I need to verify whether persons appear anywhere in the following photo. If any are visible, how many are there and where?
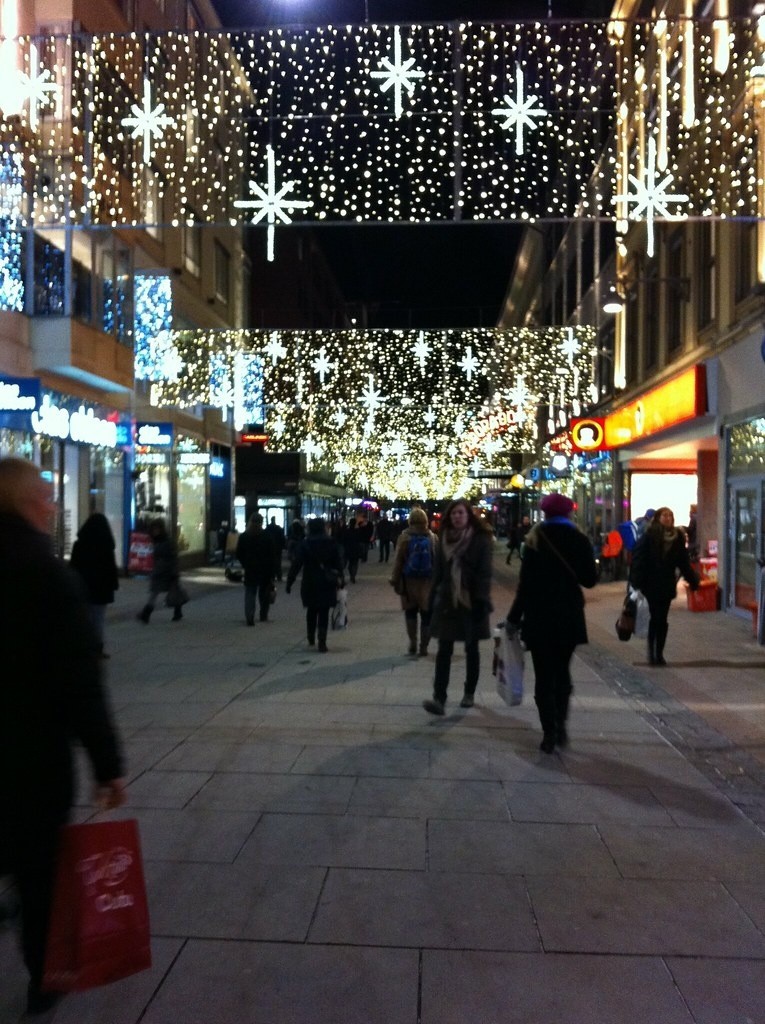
[506,493,598,752]
[70,513,120,658]
[138,517,182,625]
[630,508,697,665]
[219,503,699,652]
[0,458,130,1014]
[420,498,491,716]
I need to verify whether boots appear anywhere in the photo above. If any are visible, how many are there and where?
[533,685,572,755]
[646,619,669,667]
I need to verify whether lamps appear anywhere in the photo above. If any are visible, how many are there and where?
[602,277,690,313]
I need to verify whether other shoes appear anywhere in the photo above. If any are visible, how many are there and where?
[460,692,474,707]
[422,698,445,716]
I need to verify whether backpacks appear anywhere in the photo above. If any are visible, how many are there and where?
[402,532,434,579]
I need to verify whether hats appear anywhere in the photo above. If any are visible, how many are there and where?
[539,493,573,517]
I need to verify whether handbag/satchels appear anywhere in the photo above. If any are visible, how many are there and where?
[491,625,524,707]
[331,587,349,630]
[615,589,652,642]
[266,581,277,604]
[165,585,190,608]
[39,799,152,989]
